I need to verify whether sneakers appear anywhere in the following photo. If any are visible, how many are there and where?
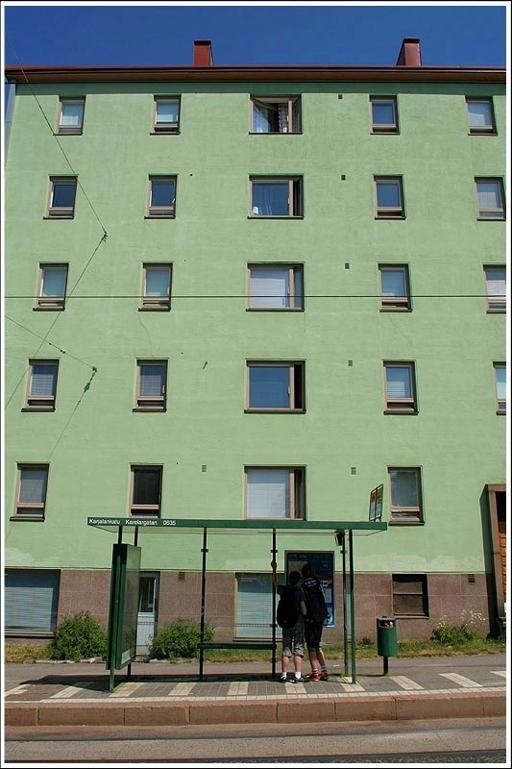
[277,674,329,683]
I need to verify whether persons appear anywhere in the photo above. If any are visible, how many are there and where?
[299,561,328,682]
[271,560,308,683]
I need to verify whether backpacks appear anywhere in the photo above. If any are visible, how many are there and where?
[303,582,328,622]
[277,587,299,629]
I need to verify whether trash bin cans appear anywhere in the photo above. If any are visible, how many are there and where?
[376,615,397,675]
